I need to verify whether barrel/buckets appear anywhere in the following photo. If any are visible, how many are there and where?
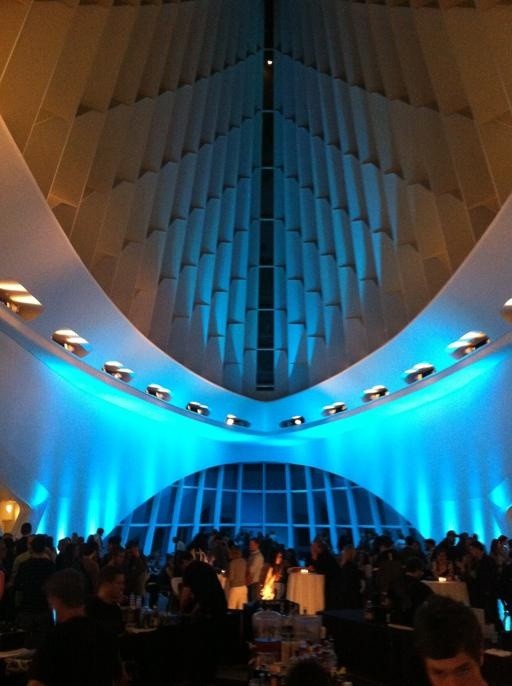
[294,615,321,642]
[253,610,282,642]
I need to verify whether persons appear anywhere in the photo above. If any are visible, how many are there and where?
[5,521,512,685]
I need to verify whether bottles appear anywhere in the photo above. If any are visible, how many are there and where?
[128,592,160,628]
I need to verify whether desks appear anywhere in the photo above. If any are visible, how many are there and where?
[423,580,470,607]
[289,573,325,615]
